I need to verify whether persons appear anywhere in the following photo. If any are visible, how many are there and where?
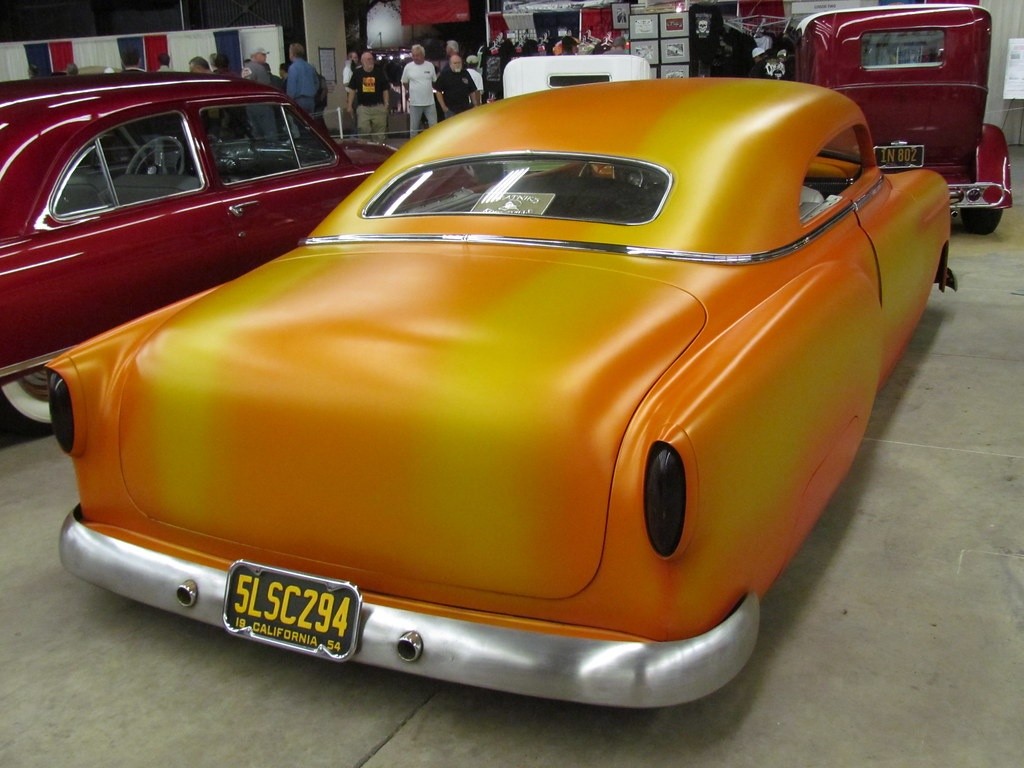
[348,52,390,144]
[121,48,145,73]
[603,37,626,54]
[29,64,40,78]
[68,65,78,75]
[190,52,246,140]
[158,54,173,72]
[240,43,328,141]
[560,37,580,55]
[435,41,478,123]
[401,45,437,139]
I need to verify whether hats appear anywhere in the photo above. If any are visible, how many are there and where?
[752,47,765,58]
[250,46,269,55]
[103,67,115,73]
[466,55,479,63]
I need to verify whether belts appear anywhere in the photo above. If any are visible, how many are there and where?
[360,101,384,107]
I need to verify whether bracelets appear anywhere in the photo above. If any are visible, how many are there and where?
[385,103,390,105]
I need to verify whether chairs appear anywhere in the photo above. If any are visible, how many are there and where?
[560,161,644,220]
[800,158,859,200]
[55,174,203,213]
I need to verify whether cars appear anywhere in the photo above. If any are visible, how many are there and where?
[788,3,1013,237]
[0,72,402,436]
[46,75,952,709]
